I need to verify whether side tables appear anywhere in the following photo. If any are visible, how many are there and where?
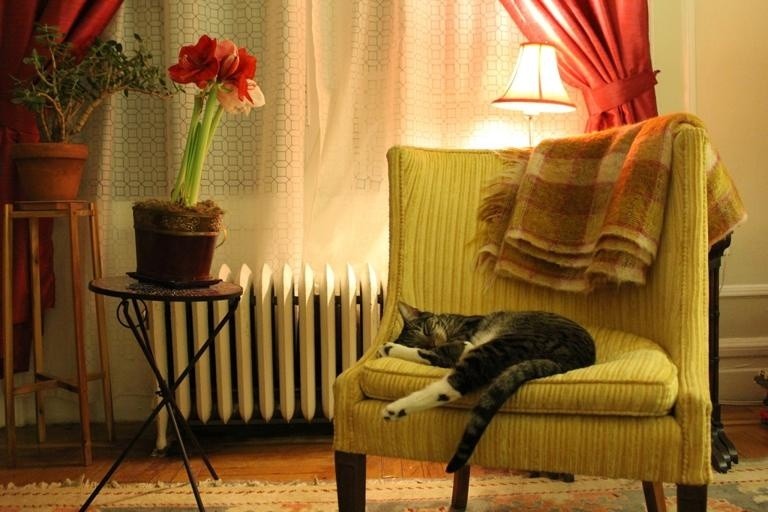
[77,276,243,512]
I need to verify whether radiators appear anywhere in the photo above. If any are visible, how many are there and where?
[146,263,387,451]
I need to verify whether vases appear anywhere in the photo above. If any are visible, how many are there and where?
[119,201,234,286]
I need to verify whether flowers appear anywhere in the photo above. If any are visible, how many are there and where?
[169,35,266,207]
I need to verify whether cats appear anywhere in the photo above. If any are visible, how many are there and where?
[376,300,596,473]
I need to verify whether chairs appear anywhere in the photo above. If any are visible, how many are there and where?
[331,122,712,512]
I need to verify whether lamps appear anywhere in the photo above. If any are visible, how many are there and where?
[491,42,576,149]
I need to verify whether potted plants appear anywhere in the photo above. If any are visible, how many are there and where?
[7,24,186,201]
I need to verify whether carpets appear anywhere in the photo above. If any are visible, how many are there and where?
[0,458,768,512]
